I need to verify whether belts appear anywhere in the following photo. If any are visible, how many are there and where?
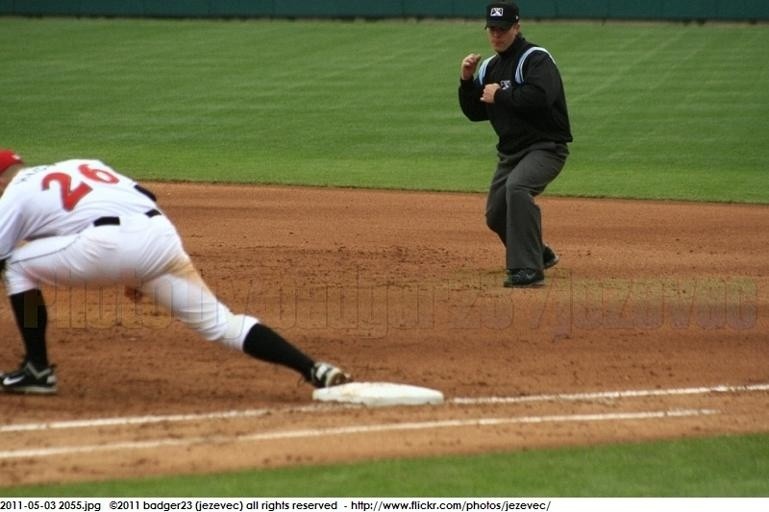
[85,208,164,230]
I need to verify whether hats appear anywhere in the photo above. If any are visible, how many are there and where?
[0,149,23,176]
[482,2,521,31]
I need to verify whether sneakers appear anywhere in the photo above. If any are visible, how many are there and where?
[308,361,353,388]
[504,243,559,275]
[502,267,545,288]
[0,361,60,394]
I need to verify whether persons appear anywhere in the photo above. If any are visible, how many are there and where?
[456,1,575,289]
[0,146,355,399]
